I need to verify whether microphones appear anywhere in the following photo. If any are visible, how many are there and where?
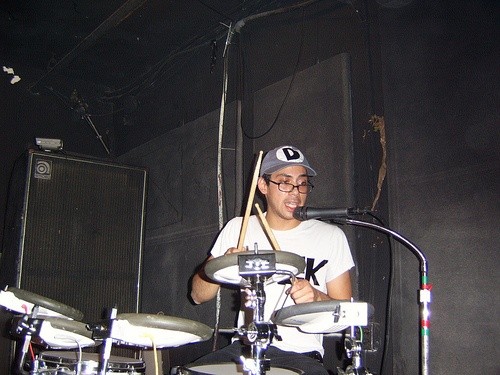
[292,206,371,221]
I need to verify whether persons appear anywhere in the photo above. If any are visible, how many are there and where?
[186,146,355,375]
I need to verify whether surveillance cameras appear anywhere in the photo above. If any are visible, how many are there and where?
[35,137,64,153]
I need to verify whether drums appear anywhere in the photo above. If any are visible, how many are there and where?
[203,250,306,288]
[36,351,146,375]
[113,313,213,348]
[0,287,84,320]
[179,362,305,375]
[11,315,103,348]
[271,301,375,333]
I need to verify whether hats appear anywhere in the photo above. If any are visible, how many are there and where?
[258,145,318,178]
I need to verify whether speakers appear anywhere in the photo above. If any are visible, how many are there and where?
[9,149,149,375]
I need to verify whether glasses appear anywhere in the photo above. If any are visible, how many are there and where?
[265,178,315,194]
